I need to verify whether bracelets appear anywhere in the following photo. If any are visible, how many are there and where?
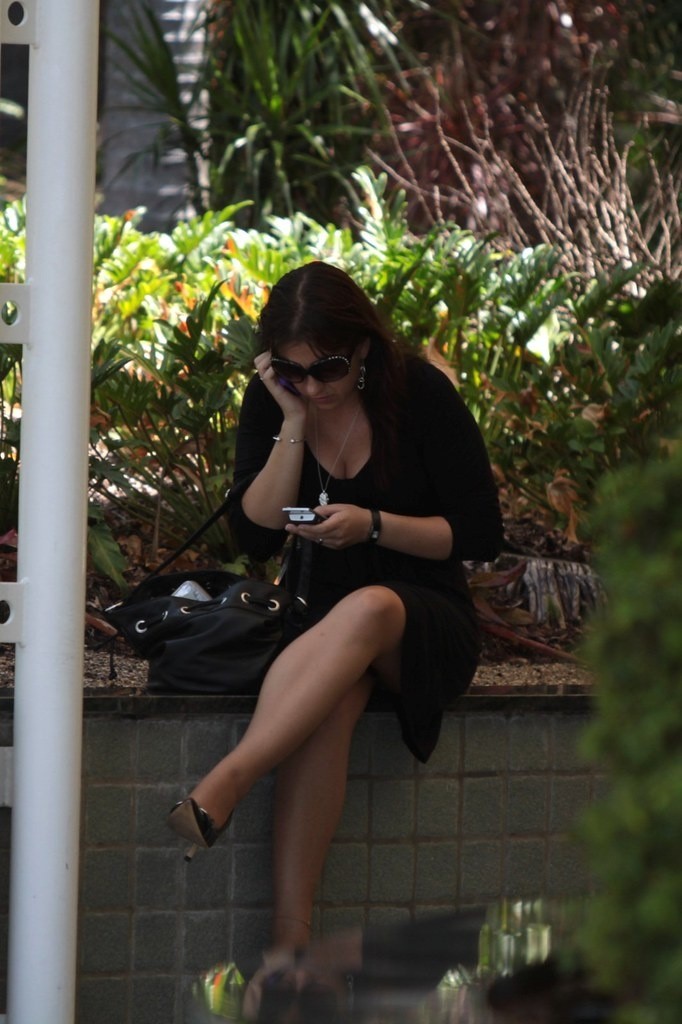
[272,435,308,444]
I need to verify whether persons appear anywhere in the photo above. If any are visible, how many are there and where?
[169,262,504,1024]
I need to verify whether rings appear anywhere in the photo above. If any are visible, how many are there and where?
[317,539,325,544]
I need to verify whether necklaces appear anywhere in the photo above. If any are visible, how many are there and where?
[313,402,362,507]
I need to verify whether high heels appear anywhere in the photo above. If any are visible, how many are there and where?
[166,797,234,863]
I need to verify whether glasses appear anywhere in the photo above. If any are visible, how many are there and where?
[270,339,361,384]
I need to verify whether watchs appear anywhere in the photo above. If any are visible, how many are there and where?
[364,507,382,552]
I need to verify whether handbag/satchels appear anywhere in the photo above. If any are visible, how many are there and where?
[102,472,321,695]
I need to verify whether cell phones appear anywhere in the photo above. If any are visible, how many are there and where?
[282,507,329,525]
[277,374,302,397]
